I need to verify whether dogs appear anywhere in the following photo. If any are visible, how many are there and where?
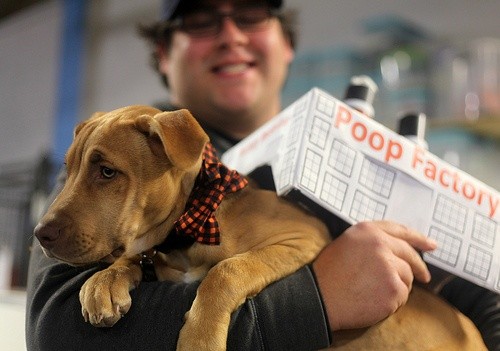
[31,103,489,351]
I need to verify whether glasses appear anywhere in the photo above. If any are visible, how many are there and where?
[173,6,278,36]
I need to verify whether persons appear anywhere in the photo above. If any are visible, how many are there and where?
[25,0,500,351]
